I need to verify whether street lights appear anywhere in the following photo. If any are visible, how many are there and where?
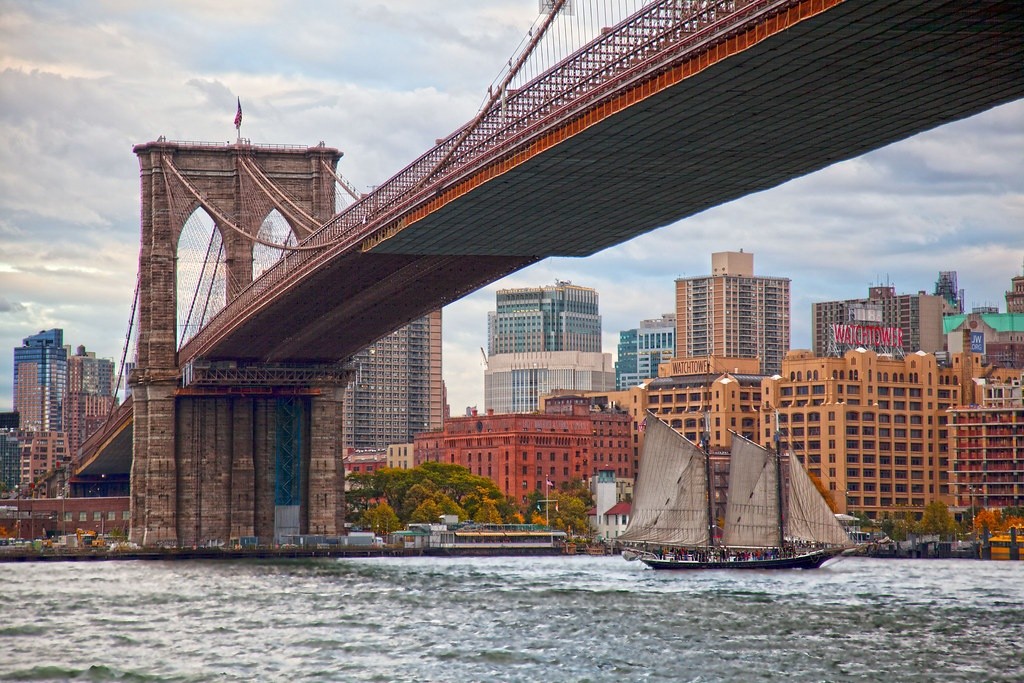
[967,485,978,529]
[15,483,20,538]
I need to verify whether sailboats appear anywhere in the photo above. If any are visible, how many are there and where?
[607,353,895,570]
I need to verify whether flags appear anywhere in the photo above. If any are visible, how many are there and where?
[234,101,242,129]
[547,480,553,486]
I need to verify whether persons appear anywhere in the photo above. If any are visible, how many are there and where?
[659,546,779,562]
[783,540,823,558]
